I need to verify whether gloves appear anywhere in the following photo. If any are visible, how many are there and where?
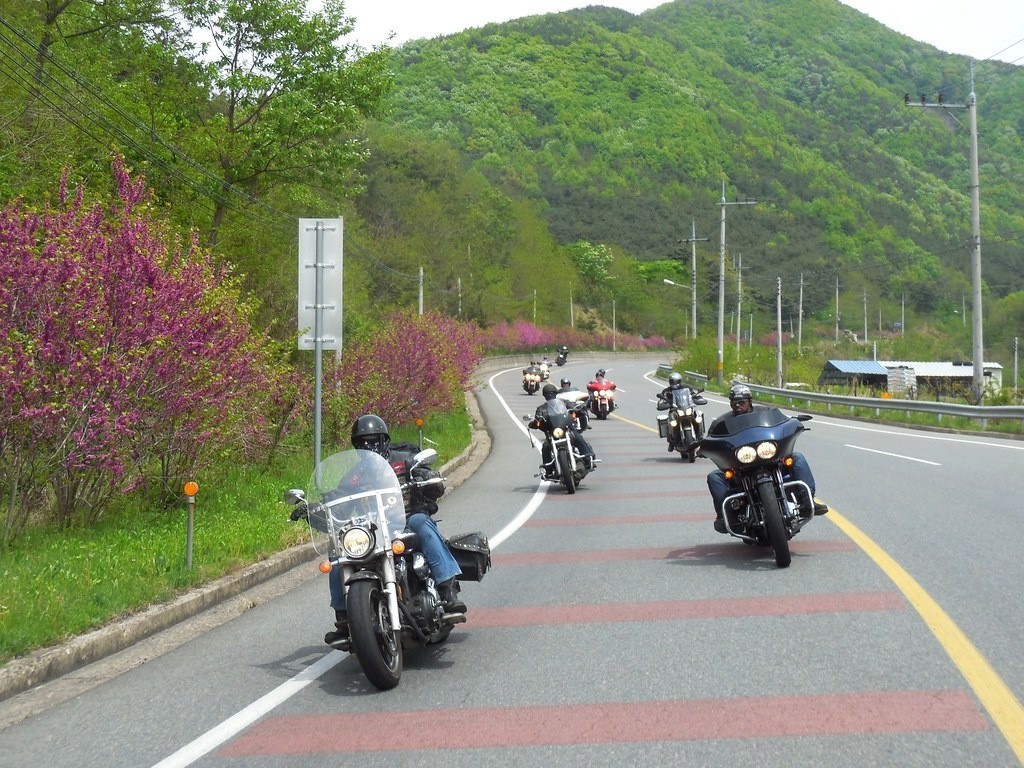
[425,471,441,481]
[290,504,308,522]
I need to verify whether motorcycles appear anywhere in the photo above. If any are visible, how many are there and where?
[521,399,602,494]
[584,376,618,420]
[283,419,493,691]
[689,408,818,568]
[554,348,570,366]
[553,386,594,436]
[522,369,544,395]
[655,386,708,463]
[536,361,553,382]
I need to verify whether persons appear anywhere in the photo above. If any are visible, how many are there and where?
[561,346,569,363]
[705,384,828,533]
[542,356,552,367]
[535,361,543,372]
[658,370,712,460]
[290,415,468,651]
[528,383,597,479]
[586,367,618,409]
[557,378,593,430]
[526,360,540,373]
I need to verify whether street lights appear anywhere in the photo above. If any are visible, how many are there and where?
[661,278,697,344]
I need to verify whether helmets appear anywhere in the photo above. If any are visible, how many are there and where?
[728,385,753,401]
[543,356,547,360]
[596,372,605,378]
[599,369,606,374]
[531,361,536,365]
[562,345,567,350]
[668,373,683,390]
[560,377,572,387]
[350,414,391,447]
[542,383,559,396]
[536,361,542,365]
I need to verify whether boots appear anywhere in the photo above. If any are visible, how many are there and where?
[667,439,675,452]
[540,468,560,481]
[438,576,467,613]
[798,493,828,518]
[324,610,350,644]
[714,506,745,534]
[583,457,597,470]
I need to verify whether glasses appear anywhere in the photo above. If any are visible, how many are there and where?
[732,400,748,405]
